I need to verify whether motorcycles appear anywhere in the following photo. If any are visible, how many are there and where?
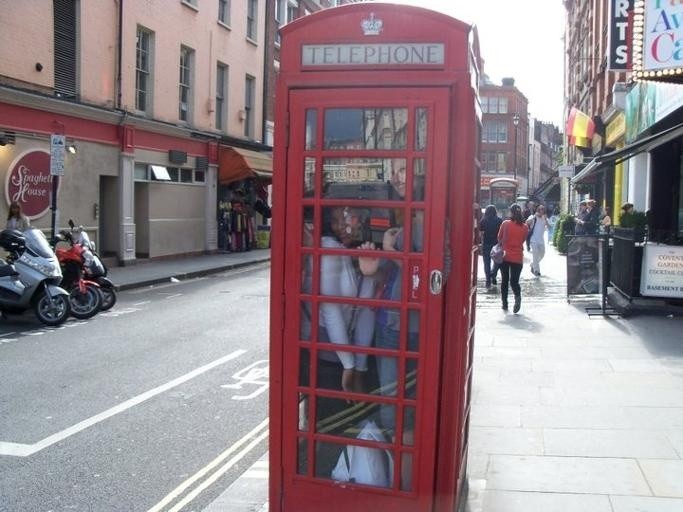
[0,218,117,326]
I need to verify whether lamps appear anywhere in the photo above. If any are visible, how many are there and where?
[65,143,77,154]
[0,132,16,146]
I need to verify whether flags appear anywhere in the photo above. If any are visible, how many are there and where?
[566,134,592,151]
[565,106,598,140]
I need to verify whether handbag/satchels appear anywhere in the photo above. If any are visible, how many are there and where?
[488,242,508,266]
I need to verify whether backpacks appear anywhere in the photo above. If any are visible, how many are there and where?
[330,421,396,490]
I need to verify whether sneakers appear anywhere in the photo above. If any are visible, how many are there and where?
[501,303,509,311]
[529,263,542,277]
[513,299,522,314]
[484,276,498,290]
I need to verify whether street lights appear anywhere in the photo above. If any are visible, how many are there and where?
[512,111,521,180]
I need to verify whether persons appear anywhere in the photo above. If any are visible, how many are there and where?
[573,201,589,235]
[598,205,611,229]
[524,202,552,278]
[521,199,535,252]
[298,183,381,474]
[579,199,602,236]
[371,140,452,495]
[478,203,503,289]
[496,203,529,313]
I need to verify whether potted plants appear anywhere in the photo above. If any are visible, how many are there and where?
[577,184,591,194]
